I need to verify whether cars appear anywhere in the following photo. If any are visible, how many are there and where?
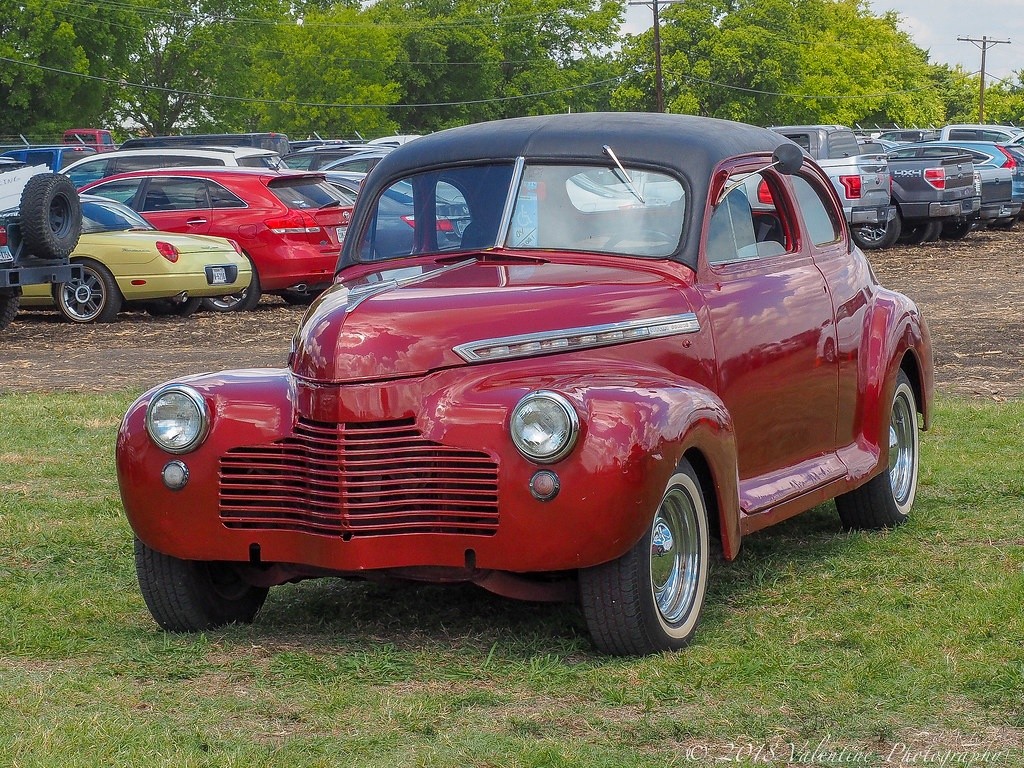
[1,120,1024,325]
[116,109,936,659]
